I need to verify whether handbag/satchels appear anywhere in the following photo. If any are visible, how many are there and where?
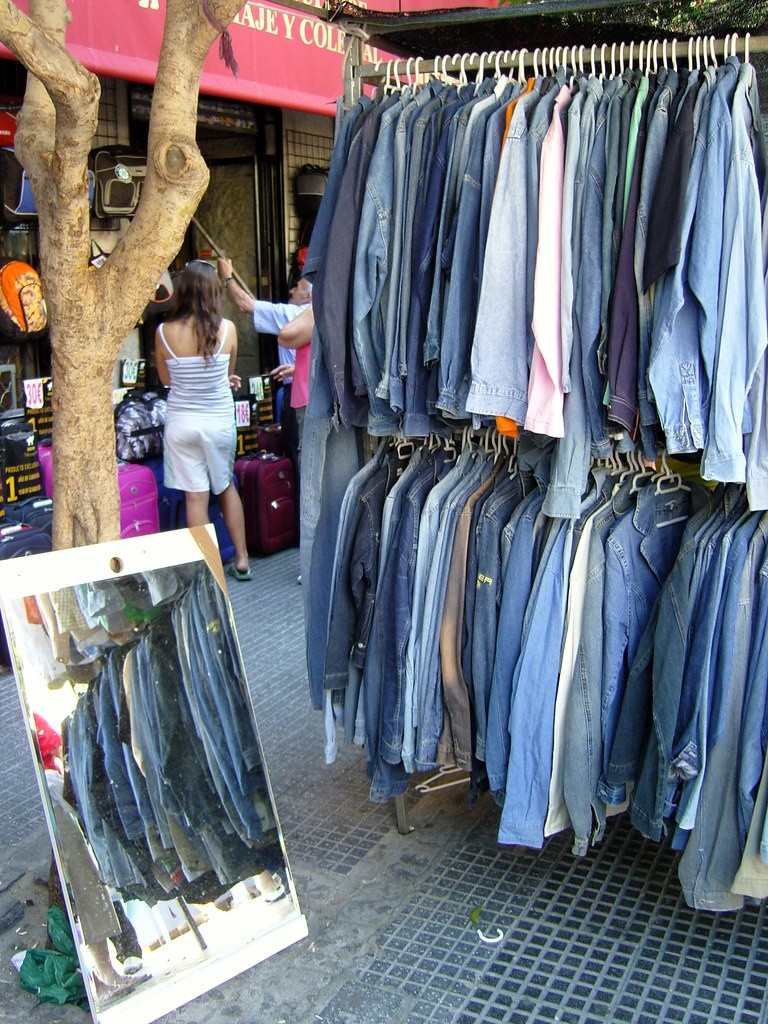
[113,386,167,462]
[0,108,148,339]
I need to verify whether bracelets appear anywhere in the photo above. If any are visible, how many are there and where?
[225,277,233,282]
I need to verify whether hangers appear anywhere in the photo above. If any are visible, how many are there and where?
[383,423,700,531]
[367,32,752,101]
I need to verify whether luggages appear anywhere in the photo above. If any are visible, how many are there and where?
[1,449,298,666]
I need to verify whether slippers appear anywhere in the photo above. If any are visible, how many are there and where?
[227,562,251,579]
[93,975,134,1007]
[115,973,153,987]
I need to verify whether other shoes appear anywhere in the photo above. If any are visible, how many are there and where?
[123,955,143,973]
[263,882,285,901]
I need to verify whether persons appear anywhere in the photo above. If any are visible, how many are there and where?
[217,256,313,459]
[31,714,143,975]
[50,804,152,1004]
[270,307,316,474]
[156,259,251,579]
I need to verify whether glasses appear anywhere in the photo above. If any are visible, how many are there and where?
[186,260,216,273]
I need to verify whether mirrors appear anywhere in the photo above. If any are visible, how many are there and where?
[0,523,311,1024]
